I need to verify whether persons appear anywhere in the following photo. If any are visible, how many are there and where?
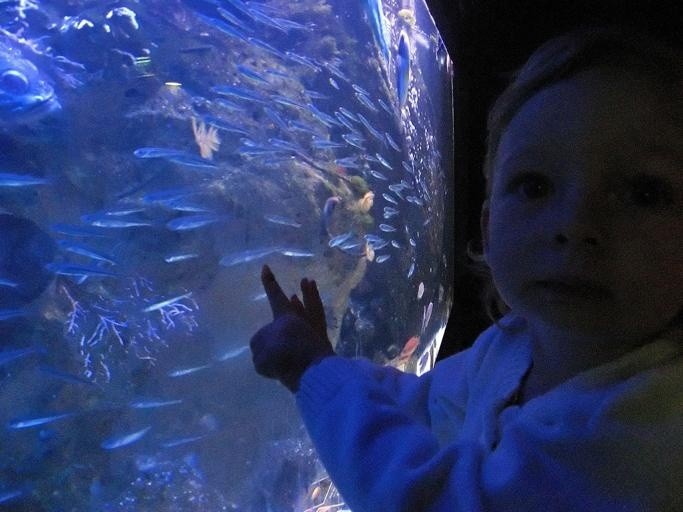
[251,27,682,511]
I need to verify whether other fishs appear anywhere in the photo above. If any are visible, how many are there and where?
[1,1,450,512]
